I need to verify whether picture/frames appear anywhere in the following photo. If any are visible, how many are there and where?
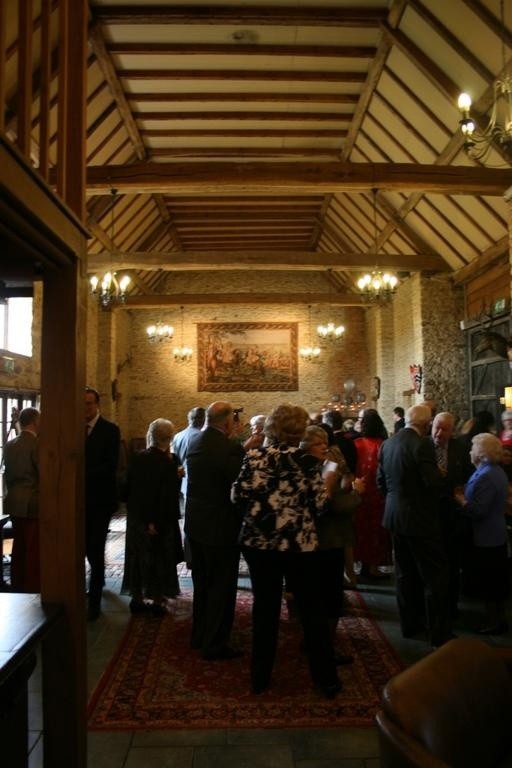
[196,320,299,392]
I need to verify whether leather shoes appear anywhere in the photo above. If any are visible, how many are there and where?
[86,601,101,626]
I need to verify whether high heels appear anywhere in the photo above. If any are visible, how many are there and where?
[149,605,168,618]
[130,599,152,616]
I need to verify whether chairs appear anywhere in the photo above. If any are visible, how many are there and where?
[371,633,512,767]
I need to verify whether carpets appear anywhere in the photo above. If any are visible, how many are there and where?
[81,585,406,733]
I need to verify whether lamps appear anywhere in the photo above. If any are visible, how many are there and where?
[144,268,176,343]
[456,0,512,163]
[316,267,346,342]
[86,190,131,308]
[354,188,400,305]
[298,302,320,360]
[172,305,194,363]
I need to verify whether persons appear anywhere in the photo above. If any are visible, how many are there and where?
[451,431,508,635]
[392,407,406,433]
[430,411,469,486]
[169,407,210,464]
[241,414,267,451]
[78,387,122,613]
[301,425,354,490]
[342,419,363,434]
[391,406,406,435]
[231,403,346,702]
[242,414,266,449]
[350,409,393,588]
[184,401,248,662]
[500,409,512,445]
[0,408,41,593]
[322,409,358,473]
[120,419,186,623]
[498,444,512,482]
[375,406,459,647]
